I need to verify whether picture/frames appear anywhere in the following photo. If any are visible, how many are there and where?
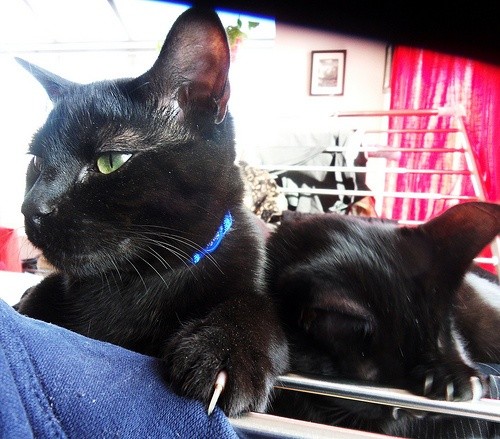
[309,48,347,96]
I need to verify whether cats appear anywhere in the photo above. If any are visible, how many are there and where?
[12,5,500,439]
